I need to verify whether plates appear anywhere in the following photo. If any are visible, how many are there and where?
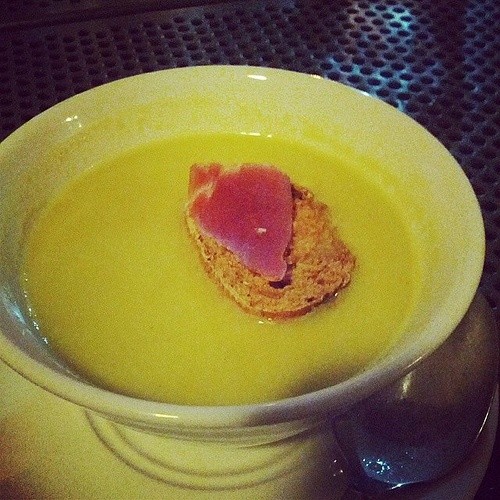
[1,357,498,498]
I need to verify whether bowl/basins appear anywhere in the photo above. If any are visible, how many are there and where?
[1,65,486,446]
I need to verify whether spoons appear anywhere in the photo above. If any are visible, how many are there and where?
[330,292,498,498]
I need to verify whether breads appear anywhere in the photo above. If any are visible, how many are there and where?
[185,182,358,316]
